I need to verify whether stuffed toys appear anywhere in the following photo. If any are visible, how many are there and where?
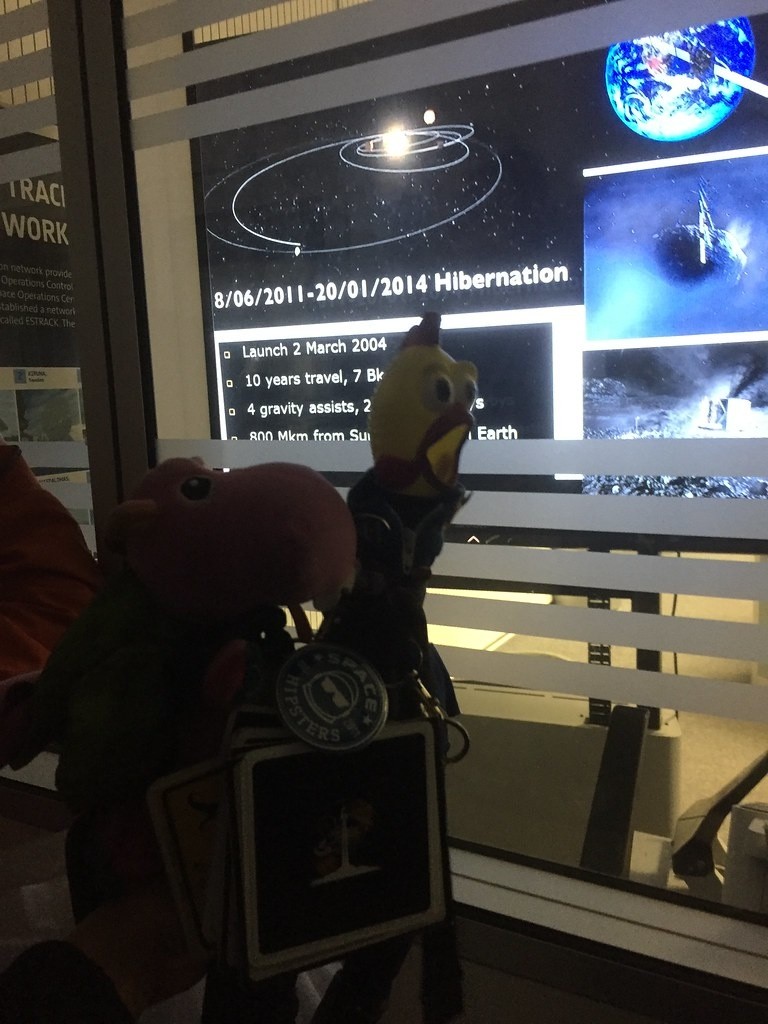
[2,312,480,1024]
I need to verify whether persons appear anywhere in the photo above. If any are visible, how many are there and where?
[318,786,383,877]
